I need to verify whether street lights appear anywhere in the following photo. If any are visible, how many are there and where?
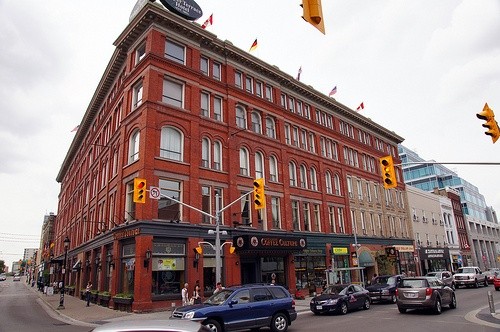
[57,235,71,311]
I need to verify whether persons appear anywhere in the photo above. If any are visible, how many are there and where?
[86,281,93,308]
[182,283,189,306]
[214,282,226,302]
[194,280,201,305]
[321,278,327,292]
[372,274,377,284]
[309,280,315,297]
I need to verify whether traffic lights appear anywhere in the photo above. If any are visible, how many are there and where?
[133,178,147,205]
[230,246,236,255]
[253,178,265,209]
[380,155,398,189]
[476,102,500,143]
[193,246,203,255]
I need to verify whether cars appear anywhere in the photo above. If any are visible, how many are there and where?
[481,267,500,291]
[0,273,6,281]
[13,273,20,281]
[88,318,211,332]
[309,283,373,316]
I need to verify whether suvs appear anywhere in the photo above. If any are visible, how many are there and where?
[394,276,457,315]
[452,266,489,289]
[365,273,407,303]
[168,283,297,332]
[425,271,457,290]
